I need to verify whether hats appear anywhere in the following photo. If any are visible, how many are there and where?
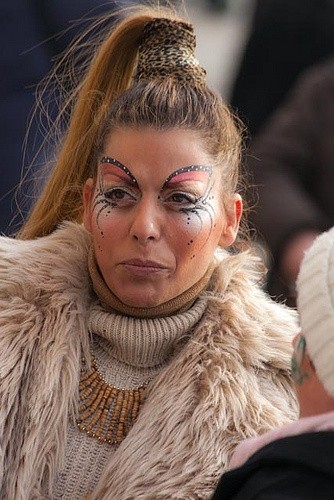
[292,226,334,398]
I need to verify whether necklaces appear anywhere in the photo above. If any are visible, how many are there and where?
[72,352,148,448]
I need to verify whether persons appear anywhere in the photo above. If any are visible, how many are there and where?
[0,0,115,239]
[243,54,334,310]
[113,0,334,144]
[213,228,334,500]
[0,4,304,500]
